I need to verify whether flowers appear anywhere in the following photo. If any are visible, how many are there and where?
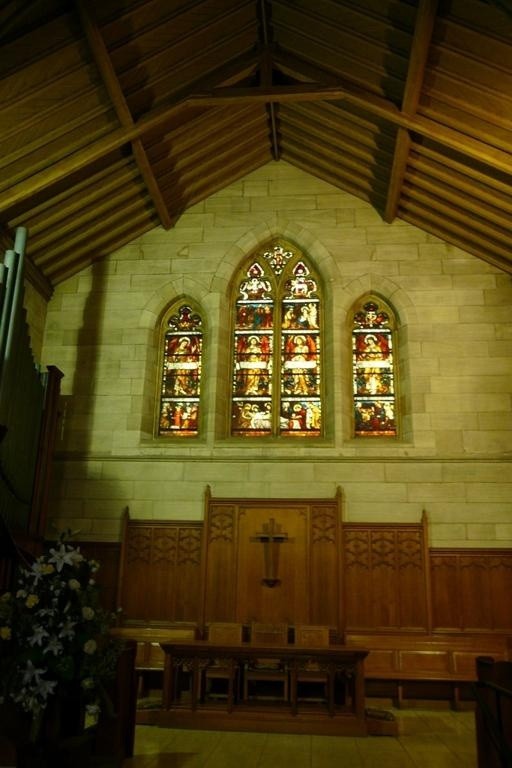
[0,526,134,745]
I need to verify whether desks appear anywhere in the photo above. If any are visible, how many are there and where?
[159,639,370,738]
